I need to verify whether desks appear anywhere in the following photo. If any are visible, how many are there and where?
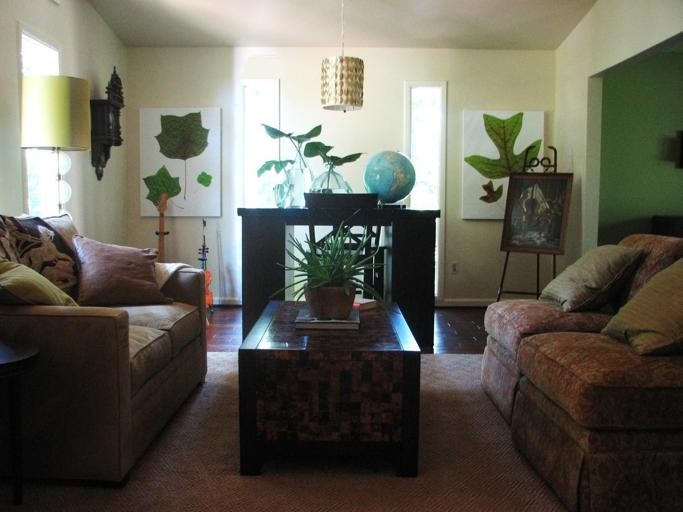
[238,208,441,351]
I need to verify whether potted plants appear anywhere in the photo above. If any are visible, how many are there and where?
[305,143,367,193]
[256,123,322,208]
[266,221,387,320]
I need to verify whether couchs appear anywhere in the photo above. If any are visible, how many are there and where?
[511,331,683,512]
[0,214,207,488]
[480,235,683,427]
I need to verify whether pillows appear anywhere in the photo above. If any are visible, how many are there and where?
[603,258,683,353]
[0,214,78,301]
[0,260,78,306]
[538,245,643,312]
[73,235,172,306]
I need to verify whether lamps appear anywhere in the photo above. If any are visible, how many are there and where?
[20,76,89,210]
[320,56,363,111]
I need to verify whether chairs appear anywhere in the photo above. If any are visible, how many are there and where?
[303,193,378,297]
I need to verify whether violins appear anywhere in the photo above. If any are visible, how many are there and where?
[197,243,213,308]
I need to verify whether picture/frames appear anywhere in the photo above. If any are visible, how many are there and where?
[460,110,545,220]
[500,172,573,255]
[140,106,221,217]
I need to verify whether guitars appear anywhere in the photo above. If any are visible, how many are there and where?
[158,193,168,262]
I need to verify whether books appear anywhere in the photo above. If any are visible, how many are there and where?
[295,307,362,330]
[352,296,376,312]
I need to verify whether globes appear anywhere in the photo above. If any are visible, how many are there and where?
[364,151,417,203]
